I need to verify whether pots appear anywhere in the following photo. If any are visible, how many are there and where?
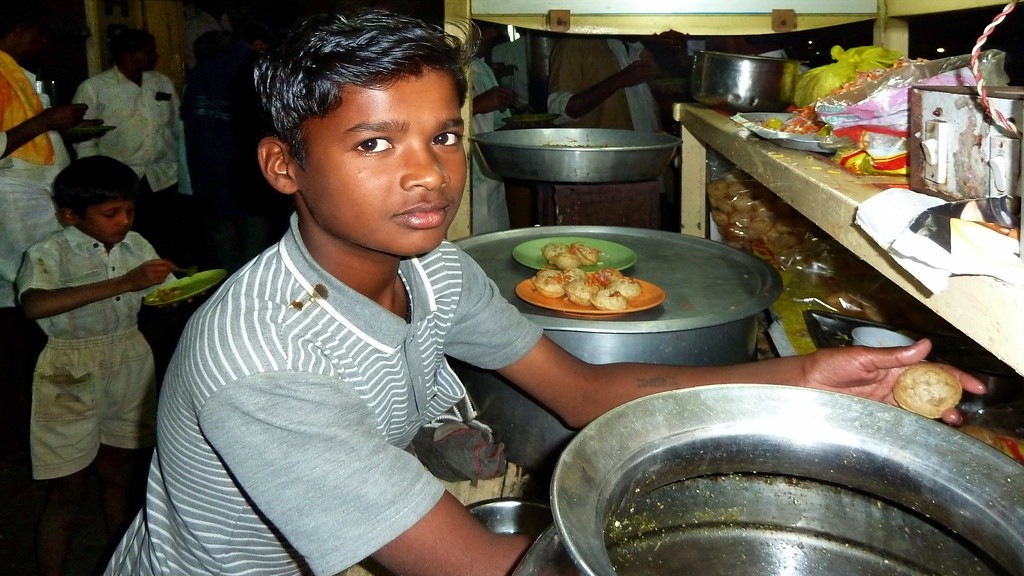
[449,224,788,491]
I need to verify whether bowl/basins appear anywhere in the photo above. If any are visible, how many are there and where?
[471,500,554,535]
[690,48,802,113]
[851,325,917,348]
[936,346,1024,403]
[469,127,684,181]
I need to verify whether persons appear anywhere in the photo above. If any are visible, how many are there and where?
[468,17,545,237]
[184,7,268,74]
[0,0,106,520]
[15,157,205,576]
[106,5,986,576]
[187,32,293,272]
[70,29,191,259]
[655,33,763,232]
[547,36,692,226]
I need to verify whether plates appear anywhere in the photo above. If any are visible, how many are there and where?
[144,269,229,305]
[648,78,684,87]
[66,125,117,134]
[513,238,638,275]
[501,113,560,125]
[517,274,666,314]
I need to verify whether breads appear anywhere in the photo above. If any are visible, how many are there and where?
[532,241,642,311]
[705,178,823,257]
[892,362,963,419]
[824,288,937,327]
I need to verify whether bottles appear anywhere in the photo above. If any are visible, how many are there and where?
[511,381,1024,576]
